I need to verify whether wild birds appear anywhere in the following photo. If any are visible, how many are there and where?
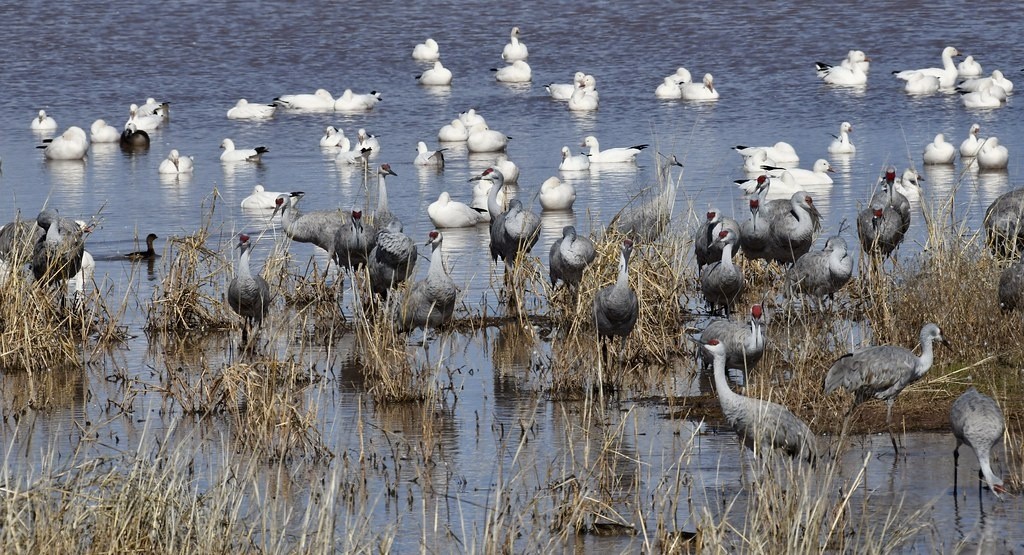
[273,88,383,113]
[36,126,89,161]
[489,27,533,83]
[30,110,57,130]
[90,120,121,143]
[124,98,171,131]
[117,123,151,150]
[269,125,457,337]
[411,38,453,85]
[655,68,720,100]
[543,72,600,110]
[226,99,278,119]
[413,108,685,412]
[0,138,307,353]
[693,47,1024,514]
